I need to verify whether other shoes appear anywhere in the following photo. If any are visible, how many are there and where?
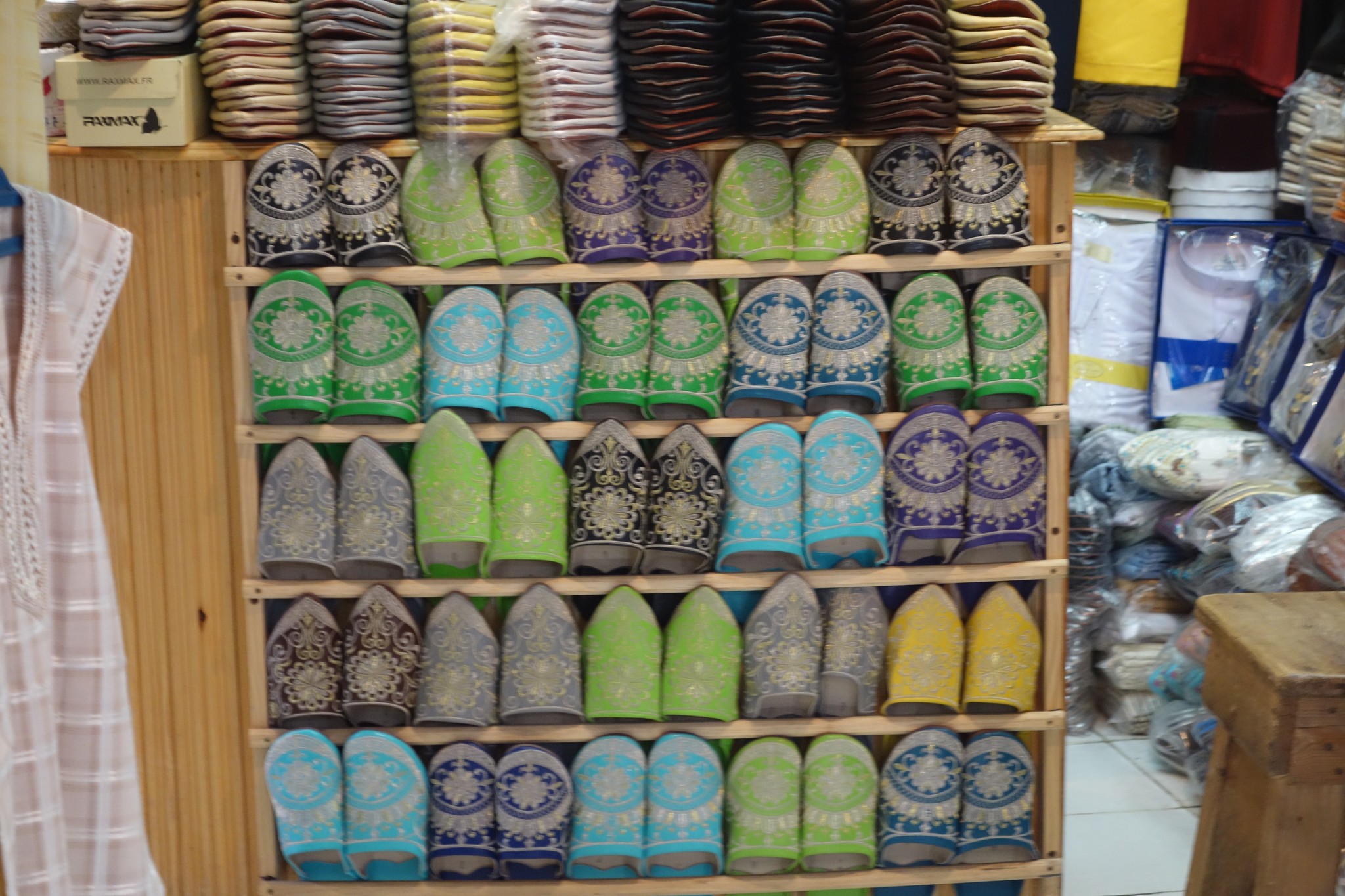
[72,0,1057,150]
[235,124,1049,896]
[1062,417,1325,785]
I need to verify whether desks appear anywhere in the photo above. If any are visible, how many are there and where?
[1184,590,1345,896]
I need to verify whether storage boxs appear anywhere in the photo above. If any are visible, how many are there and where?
[37,2,81,43]
[55,50,208,147]
[39,47,73,137]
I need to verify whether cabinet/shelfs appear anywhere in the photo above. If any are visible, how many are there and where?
[49,106,1108,896]
